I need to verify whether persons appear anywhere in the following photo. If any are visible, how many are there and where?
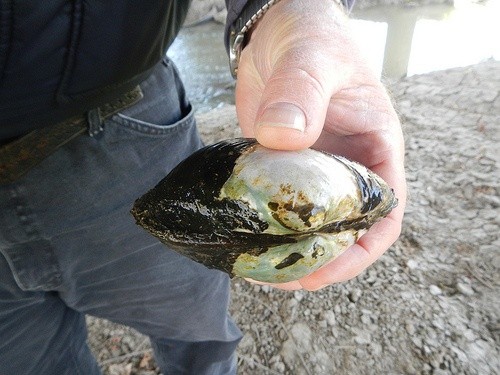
[0,0,405,375]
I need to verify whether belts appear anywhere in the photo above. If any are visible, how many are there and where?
[0,83,145,191]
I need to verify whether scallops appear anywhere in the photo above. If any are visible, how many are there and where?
[130,137,398,283]
